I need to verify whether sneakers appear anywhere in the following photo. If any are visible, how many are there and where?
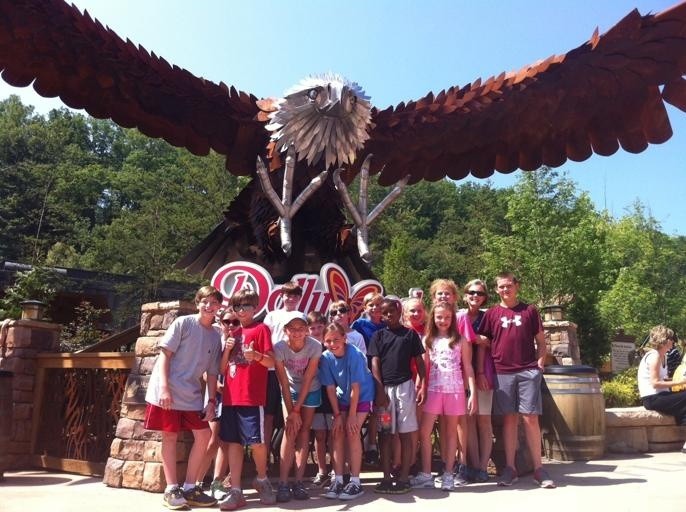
[162,488,189,510]
[373,479,411,494]
[497,466,518,487]
[276,485,291,503]
[252,477,276,504]
[311,473,365,499]
[180,486,217,507]
[410,472,436,489]
[219,489,246,510]
[532,468,555,488]
[441,464,488,490]
[293,483,309,500]
[210,481,227,500]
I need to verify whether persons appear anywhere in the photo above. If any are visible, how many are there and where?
[637,325,686,455]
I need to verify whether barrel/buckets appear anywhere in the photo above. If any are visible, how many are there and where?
[541,364,607,461]
[543,306,563,321]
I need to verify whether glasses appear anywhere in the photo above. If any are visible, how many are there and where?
[331,308,347,316]
[468,290,485,296]
[223,319,239,326]
[233,303,254,312]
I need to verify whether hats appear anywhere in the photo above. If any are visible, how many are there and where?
[283,310,308,326]
